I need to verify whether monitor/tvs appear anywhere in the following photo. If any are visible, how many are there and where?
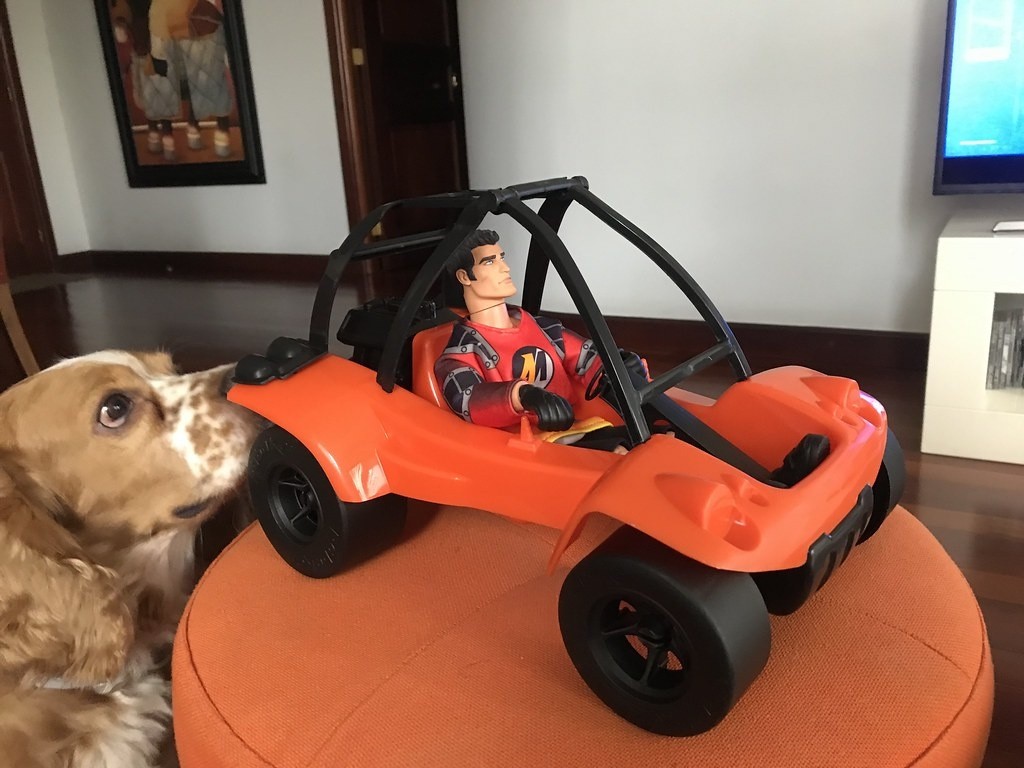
[932,0,1024,196]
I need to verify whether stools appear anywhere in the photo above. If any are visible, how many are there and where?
[172,498,996,768]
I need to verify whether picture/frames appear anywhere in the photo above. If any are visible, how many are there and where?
[94,0,267,188]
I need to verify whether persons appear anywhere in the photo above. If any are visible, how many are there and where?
[435,229,648,433]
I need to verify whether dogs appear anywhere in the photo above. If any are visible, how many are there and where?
[1,343,276,768]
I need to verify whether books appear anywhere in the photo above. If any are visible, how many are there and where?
[987,308,1024,389]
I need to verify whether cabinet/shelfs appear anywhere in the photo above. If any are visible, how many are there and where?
[921,206,1024,465]
